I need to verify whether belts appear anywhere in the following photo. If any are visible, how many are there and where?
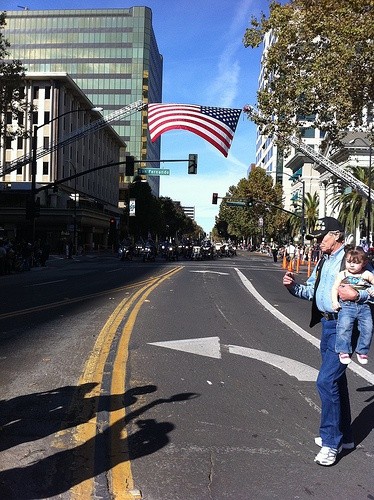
[322,313,339,321]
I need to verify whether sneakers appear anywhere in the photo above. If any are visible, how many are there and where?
[356,352,368,364]
[339,352,351,364]
[314,445,342,466]
[314,437,354,449]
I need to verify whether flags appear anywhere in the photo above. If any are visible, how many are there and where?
[148,103,242,159]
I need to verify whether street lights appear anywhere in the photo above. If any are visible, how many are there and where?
[265,170,305,251]
[29,106,104,268]
[349,136,374,250]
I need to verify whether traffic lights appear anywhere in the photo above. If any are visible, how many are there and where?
[212,193,218,204]
[247,195,253,207]
[301,224,306,234]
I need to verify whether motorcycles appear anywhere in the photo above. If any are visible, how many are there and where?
[120,242,235,262]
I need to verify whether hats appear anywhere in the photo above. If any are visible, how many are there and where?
[305,217,345,240]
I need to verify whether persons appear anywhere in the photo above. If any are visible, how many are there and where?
[283,242,319,262]
[63,236,73,259]
[333,244,374,364]
[283,217,374,465]
[271,241,279,263]
[366,242,374,257]
[117,238,237,261]
